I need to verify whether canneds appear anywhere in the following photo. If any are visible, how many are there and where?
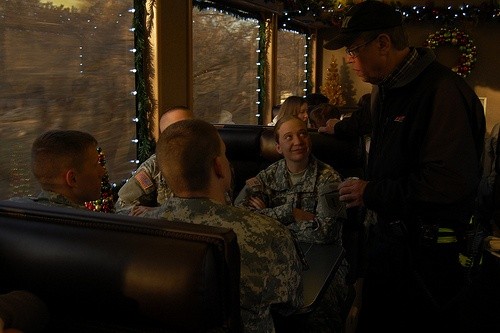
[343,177,361,209]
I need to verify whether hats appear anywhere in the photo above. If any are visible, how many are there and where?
[323,0,403,49]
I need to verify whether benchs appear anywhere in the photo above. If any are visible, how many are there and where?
[210,125,355,193]
[0,199,242,333]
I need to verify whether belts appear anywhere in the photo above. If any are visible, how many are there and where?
[375,216,475,236]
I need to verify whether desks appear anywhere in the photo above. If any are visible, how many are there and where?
[291,242,347,313]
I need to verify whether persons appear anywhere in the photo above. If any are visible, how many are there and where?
[267,92,371,180]
[111,104,234,218]
[138,118,307,333]
[4,128,106,211]
[318,0,486,333]
[233,114,351,332]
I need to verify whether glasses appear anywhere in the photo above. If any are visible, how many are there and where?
[345,36,376,57]
